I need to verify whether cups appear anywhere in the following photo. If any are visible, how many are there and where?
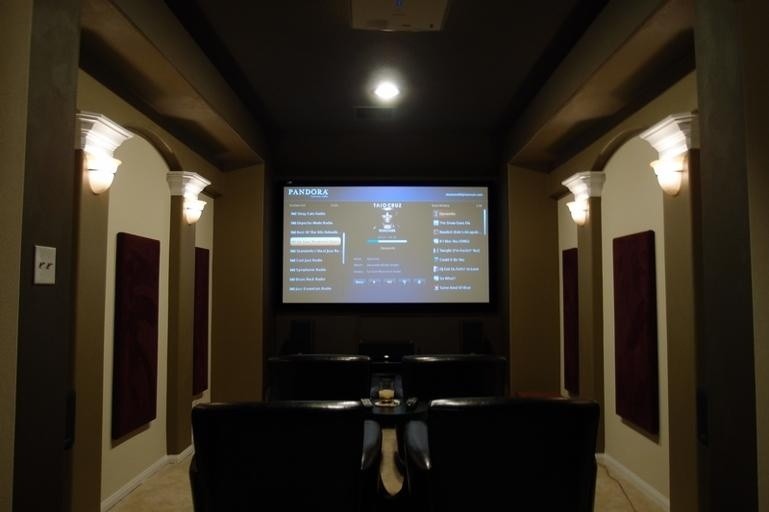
[379,389,395,405]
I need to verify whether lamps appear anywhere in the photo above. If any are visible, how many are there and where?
[87,155,207,225]
[565,157,684,227]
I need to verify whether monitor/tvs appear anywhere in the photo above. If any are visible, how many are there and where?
[273,175,495,316]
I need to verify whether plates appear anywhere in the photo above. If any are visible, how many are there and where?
[375,400,401,408]
